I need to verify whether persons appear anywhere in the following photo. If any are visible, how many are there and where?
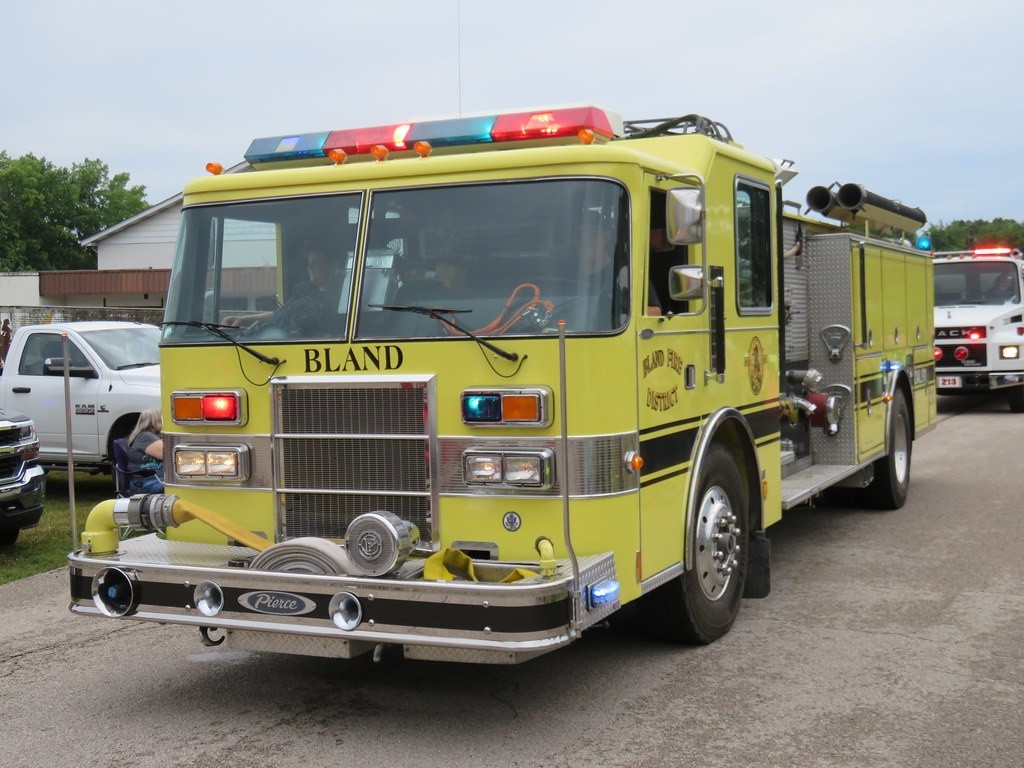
[986,272,1013,299]
[570,223,661,328]
[221,247,342,338]
[128,409,164,494]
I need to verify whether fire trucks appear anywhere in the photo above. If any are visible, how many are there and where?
[931,248,1024,411]
[65,110,955,665]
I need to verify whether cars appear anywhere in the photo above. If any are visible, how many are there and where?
[0,407,45,552]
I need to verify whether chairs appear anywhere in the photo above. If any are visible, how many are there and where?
[111,438,163,499]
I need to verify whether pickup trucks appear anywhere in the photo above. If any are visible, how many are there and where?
[1,322,163,484]
